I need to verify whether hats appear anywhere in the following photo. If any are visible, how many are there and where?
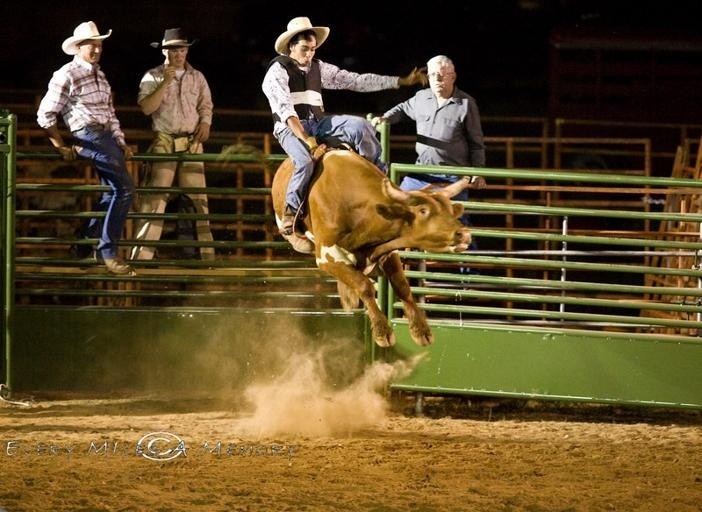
[61,21,112,55]
[151,28,196,49]
[275,15,330,55]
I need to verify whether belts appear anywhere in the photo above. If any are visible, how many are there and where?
[70,126,103,136]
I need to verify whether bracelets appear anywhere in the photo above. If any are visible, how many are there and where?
[304,136,317,150]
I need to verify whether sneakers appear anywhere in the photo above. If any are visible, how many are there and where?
[94,253,130,274]
[281,229,315,253]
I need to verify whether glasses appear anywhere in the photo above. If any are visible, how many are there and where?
[426,71,455,78]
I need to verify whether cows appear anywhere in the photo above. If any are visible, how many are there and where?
[213,142,472,348]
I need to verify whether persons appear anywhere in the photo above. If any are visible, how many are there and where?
[371,53,486,291]
[130,28,215,261]
[37,21,133,275]
[261,16,426,253]
